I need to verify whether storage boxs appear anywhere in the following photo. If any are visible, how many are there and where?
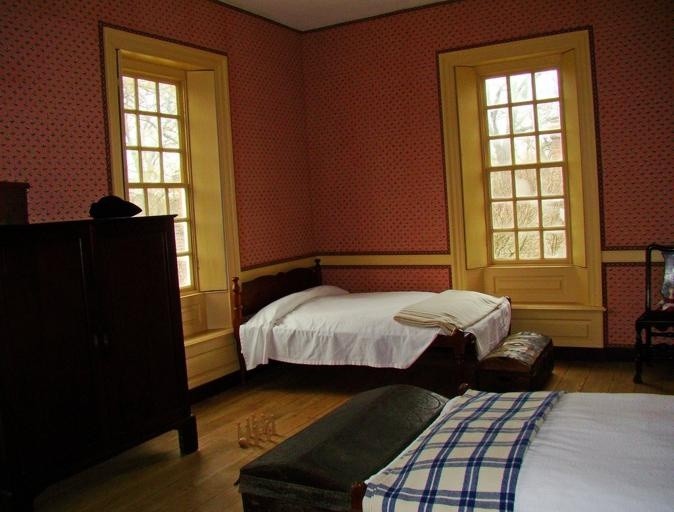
[472,329,554,394]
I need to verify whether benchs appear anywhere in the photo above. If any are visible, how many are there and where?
[235,385,449,512]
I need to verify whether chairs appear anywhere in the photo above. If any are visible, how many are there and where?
[631,242,674,385]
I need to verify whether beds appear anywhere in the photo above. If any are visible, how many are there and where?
[227,257,512,395]
[351,384,673,512]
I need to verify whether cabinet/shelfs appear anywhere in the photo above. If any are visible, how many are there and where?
[0,213,203,512]
